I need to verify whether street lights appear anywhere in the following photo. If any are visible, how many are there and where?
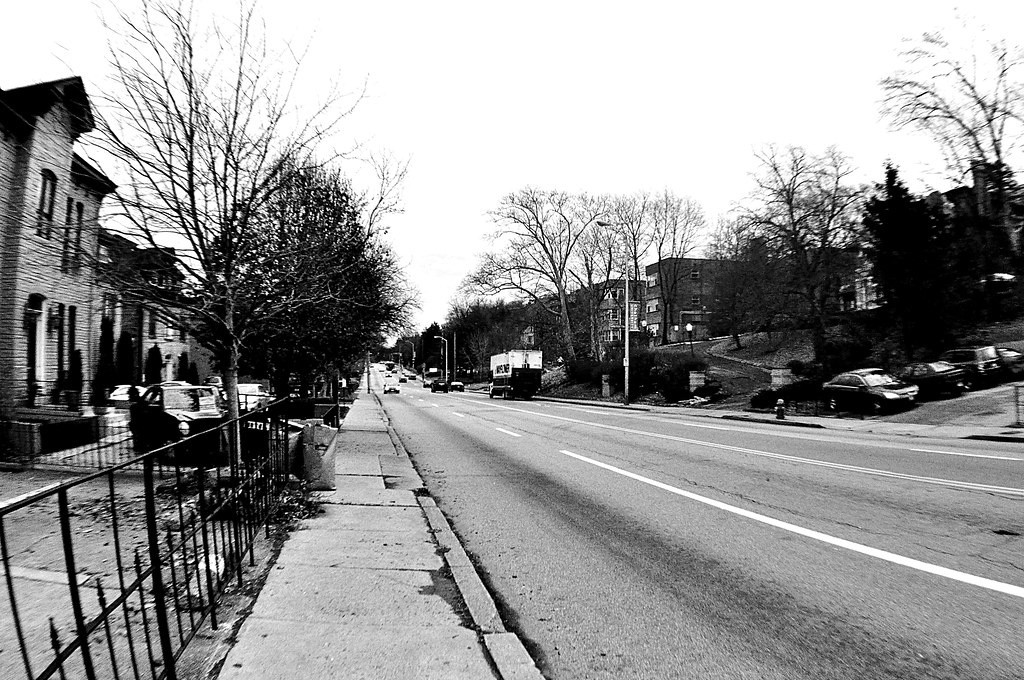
[405,340,415,368]
[596,220,629,399]
[434,334,448,383]
[685,322,694,356]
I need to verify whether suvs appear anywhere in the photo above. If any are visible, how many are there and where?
[431,380,448,393]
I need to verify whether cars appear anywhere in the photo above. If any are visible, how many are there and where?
[449,381,465,392]
[201,376,224,388]
[383,382,401,394]
[376,360,417,383]
[106,384,148,402]
[894,361,965,398]
[820,367,920,412]
[222,382,277,414]
[129,386,229,463]
[979,272,1017,294]
[145,381,193,389]
[995,347,1024,378]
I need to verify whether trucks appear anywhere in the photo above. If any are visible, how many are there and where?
[423,367,442,388]
[487,349,543,401]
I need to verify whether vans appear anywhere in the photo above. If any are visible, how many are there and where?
[937,345,1002,387]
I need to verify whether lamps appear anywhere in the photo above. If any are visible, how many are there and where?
[51,313,62,329]
